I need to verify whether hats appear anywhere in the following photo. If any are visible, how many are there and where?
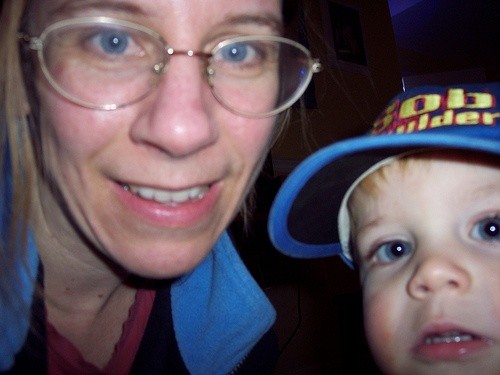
[265,70,499,267]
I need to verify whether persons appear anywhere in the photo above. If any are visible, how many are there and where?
[1,1,337,375]
[267,80,500,375]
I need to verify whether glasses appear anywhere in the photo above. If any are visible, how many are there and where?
[14,16,327,122]
